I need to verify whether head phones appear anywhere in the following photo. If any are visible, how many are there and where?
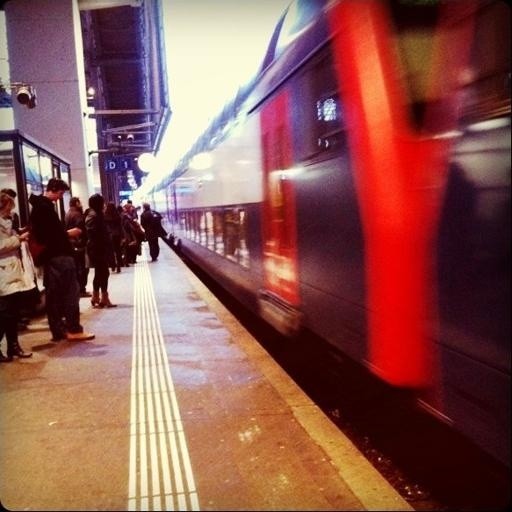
[52,178,59,191]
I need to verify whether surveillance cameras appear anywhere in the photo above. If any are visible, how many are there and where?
[127,134,134,142]
[17,85,31,104]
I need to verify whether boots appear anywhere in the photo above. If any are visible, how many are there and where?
[99,292,117,308]
[6,340,32,358]
[91,290,101,306]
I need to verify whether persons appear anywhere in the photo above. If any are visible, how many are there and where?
[65,198,92,297]
[85,193,117,307]
[1,193,46,362]
[0,189,30,235]
[104,200,167,272]
[29,178,95,340]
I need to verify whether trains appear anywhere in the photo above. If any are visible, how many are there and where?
[142,2,511,511]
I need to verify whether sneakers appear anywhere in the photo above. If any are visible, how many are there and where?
[67,330,95,340]
